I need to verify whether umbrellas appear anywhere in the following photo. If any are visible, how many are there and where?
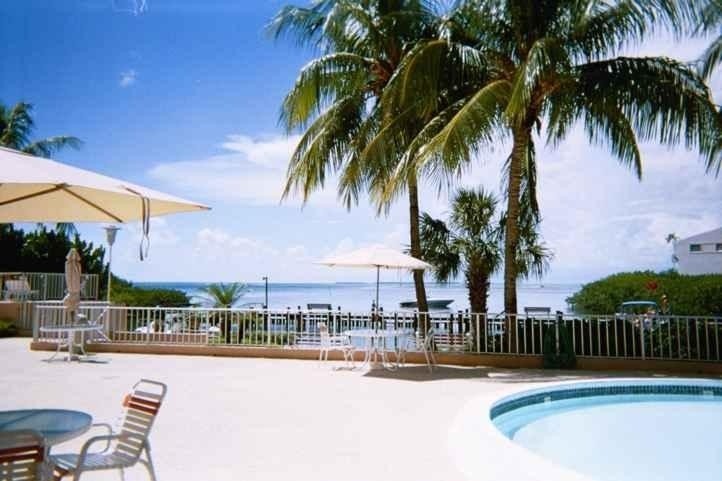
[62,248,83,326]
[315,241,434,333]
[0,148,214,227]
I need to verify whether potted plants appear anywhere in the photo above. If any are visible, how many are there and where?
[0,320,18,337]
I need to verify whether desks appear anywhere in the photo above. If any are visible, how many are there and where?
[345,329,407,372]
[0,409,92,481]
[39,325,103,363]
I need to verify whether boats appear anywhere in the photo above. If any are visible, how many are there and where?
[400,300,454,307]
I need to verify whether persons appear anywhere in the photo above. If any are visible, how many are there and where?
[659,294,674,316]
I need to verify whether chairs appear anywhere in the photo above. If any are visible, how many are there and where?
[0,429,49,481]
[6,279,39,301]
[403,328,439,372]
[46,379,167,481]
[317,322,356,371]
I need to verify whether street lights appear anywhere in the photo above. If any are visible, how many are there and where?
[104,224,121,341]
[263,276,267,309]
